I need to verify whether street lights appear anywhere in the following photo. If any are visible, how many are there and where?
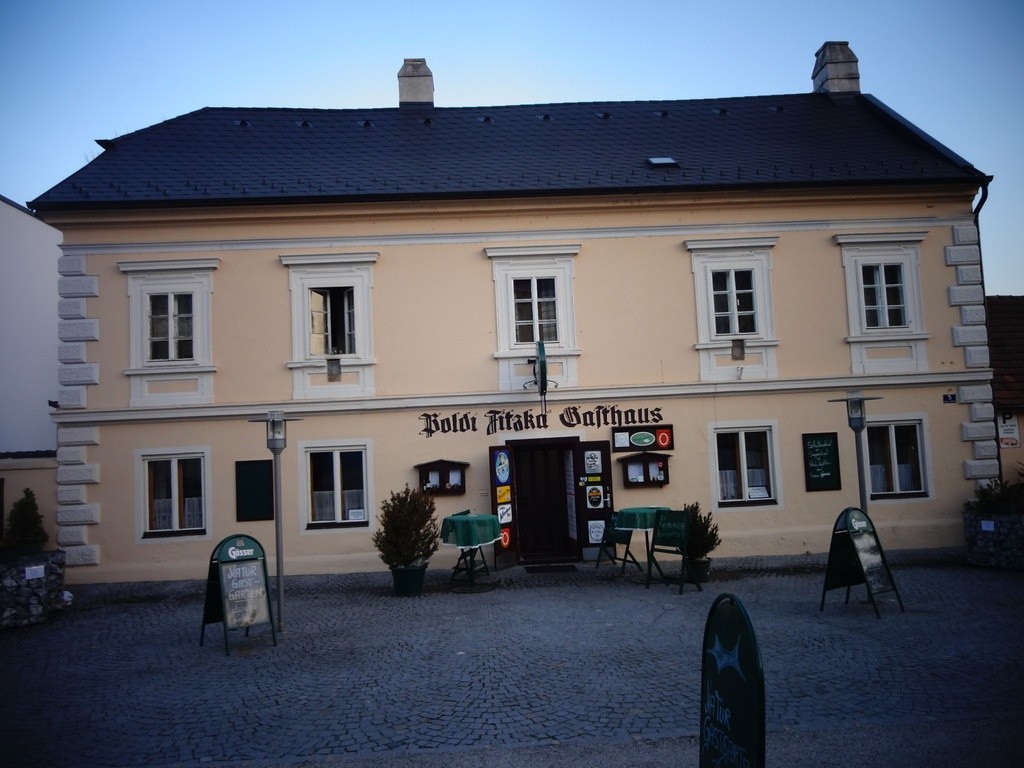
[829,395,870,516]
[248,408,304,634]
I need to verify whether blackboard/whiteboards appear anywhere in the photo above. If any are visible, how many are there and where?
[699,679,766,768]
[851,531,895,595]
[218,559,272,631]
[652,510,691,548]
[823,531,866,591]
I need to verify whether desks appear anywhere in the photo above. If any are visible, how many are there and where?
[440,514,502,588]
[617,507,671,584]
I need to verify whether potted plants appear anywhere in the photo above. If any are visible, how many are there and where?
[372,483,440,596]
[676,501,721,584]
